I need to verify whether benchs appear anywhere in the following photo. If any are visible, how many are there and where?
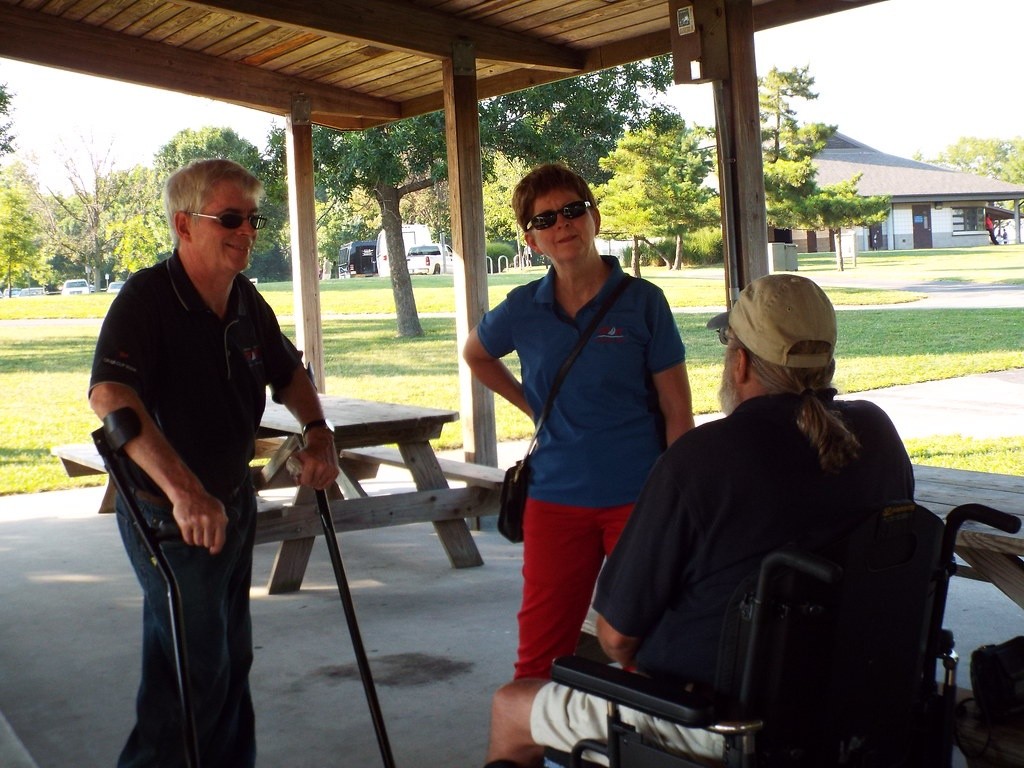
[50,443,117,513]
[340,446,507,516]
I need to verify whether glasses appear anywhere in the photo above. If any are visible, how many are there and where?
[526,200,591,230]
[184,211,267,229]
[716,328,747,351]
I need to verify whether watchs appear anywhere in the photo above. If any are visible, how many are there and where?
[302,418,335,437]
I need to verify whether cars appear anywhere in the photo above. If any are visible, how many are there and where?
[406,244,457,273]
[62,278,89,296]
[17,287,48,297]
[4,287,21,297]
[106,281,125,292]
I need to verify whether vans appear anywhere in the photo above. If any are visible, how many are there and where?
[376,224,432,275]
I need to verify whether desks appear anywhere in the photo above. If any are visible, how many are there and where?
[252,392,484,595]
[940,512,1024,609]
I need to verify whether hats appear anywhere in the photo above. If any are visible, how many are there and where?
[707,274,838,368]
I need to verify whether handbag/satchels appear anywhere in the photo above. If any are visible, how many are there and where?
[498,461,531,543]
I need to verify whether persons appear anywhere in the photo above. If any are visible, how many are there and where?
[461,165,697,680]
[488,271,914,768]
[996,229,1008,244]
[986,215,1000,245]
[88,158,342,768]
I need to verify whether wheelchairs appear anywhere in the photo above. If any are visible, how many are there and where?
[487,496,1024,767]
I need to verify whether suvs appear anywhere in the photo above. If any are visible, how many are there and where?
[335,241,377,279]
